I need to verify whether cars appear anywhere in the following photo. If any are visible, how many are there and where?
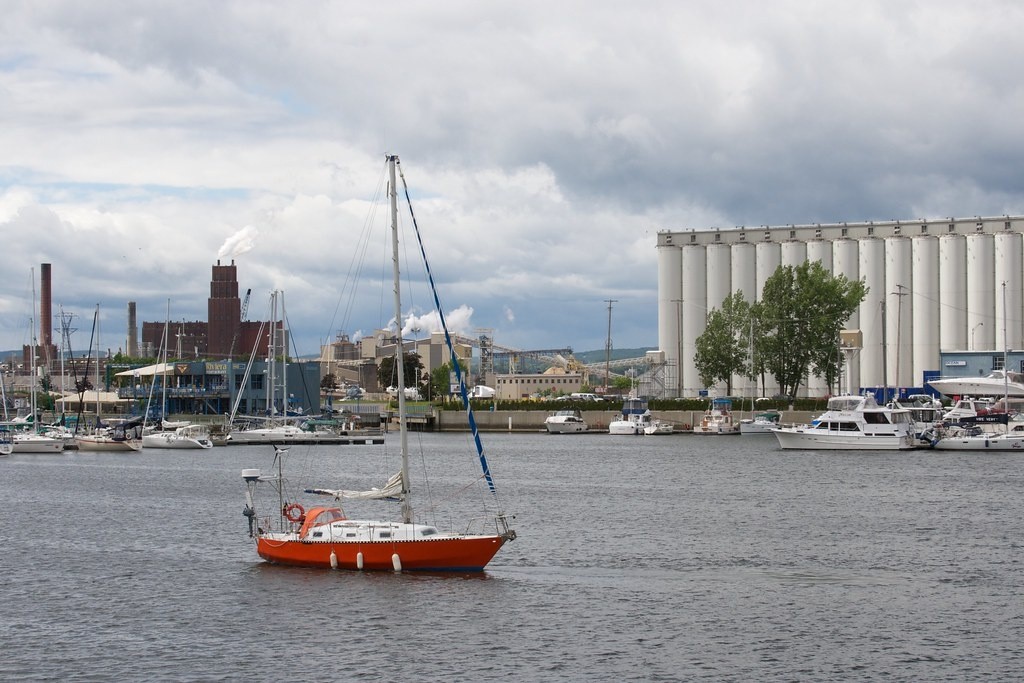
[557,393,604,402]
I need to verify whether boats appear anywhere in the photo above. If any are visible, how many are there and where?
[691,400,741,435]
[926,370,1024,401]
[609,398,652,435]
[770,396,920,450]
[644,422,673,434]
[940,399,996,421]
[544,407,588,433]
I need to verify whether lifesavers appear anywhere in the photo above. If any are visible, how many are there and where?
[126,433,131,439]
[285,504,304,522]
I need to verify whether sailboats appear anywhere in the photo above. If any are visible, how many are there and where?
[74,303,143,451]
[241,156,518,573]
[918,281,1024,450]
[880,299,942,431]
[740,318,784,435]
[228,288,321,443]
[0,268,65,454]
[142,299,214,449]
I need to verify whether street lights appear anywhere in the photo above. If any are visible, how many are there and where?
[971,322,983,350]
[414,367,418,402]
[604,299,618,393]
[65,370,71,410]
[676,299,684,397]
